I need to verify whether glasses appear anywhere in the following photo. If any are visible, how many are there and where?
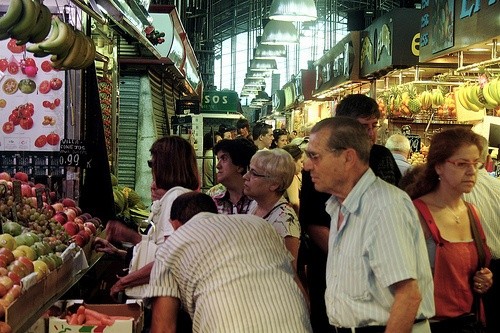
[247,166,271,177]
[297,140,308,146]
[147,160,155,168]
[448,159,484,171]
[363,123,380,134]
[305,147,345,163]
[273,129,288,133]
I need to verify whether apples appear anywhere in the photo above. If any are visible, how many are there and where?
[0,233,48,333]
[438,93,456,118]
[377,99,413,118]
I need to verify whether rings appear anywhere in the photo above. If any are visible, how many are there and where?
[478,283,481,289]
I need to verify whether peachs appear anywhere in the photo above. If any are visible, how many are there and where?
[0,170,101,246]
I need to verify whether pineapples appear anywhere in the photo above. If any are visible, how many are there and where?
[477,66,487,104]
[387,85,420,115]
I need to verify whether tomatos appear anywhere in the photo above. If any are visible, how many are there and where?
[0,39,62,147]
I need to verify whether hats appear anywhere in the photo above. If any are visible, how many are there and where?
[291,137,309,149]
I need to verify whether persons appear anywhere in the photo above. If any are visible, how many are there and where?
[241,147,301,280]
[382,133,417,176]
[210,137,258,215]
[92,137,200,312]
[203,117,309,212]
[140,189,312,333]
[410,128,492,333]
[299,93,403,333]
[304,117,435,333]
[399,162,436,199]
[464,131,500,333]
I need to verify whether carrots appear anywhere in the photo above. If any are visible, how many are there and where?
[65,305,113,326]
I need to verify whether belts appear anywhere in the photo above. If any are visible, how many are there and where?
[334,317,425,333]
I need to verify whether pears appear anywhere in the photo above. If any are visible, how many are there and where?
[0,217,63,270]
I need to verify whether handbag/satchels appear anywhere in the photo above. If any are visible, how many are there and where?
[125,185,187,298]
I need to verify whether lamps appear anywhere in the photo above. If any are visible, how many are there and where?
[240,20,298,96]
[268,0,318,22]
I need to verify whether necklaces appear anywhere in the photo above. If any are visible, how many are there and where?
[442,198,461,224]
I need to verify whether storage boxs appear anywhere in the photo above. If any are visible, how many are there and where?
[49,304,144,333]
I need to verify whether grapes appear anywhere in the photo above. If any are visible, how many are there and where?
[0,181,80,257]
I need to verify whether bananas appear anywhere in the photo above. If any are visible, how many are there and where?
[458,76,500,111]
[0,0,52,46]
[420,89,444,107]
[26,16,95,71]
